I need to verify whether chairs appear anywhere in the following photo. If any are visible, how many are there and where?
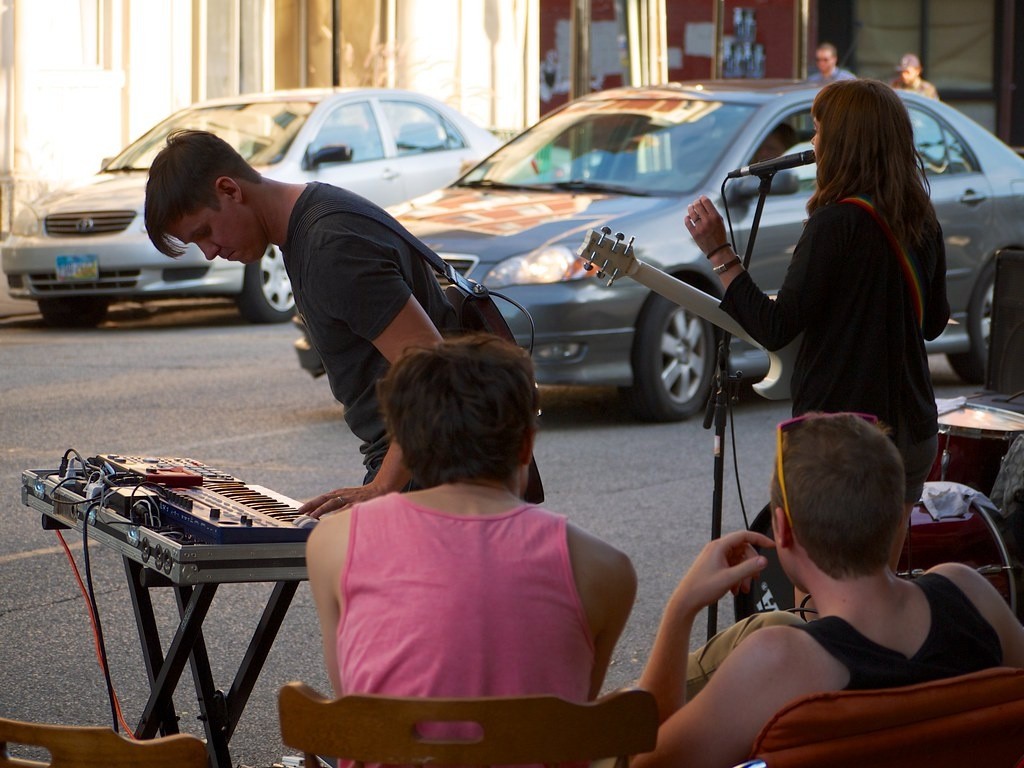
[396,122,441,150]
[319,120,366,155]
[751,668,1023,768]
[0,713,214,768]
[276,680,659,768]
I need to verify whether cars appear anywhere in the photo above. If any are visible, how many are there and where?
[1,86,570,331]
[290,79,1024,426]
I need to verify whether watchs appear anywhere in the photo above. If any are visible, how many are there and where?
[712,255,742,274]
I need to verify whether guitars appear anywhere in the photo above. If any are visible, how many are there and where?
[575,225,811,400]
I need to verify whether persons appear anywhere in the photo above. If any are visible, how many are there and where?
[623,412,1024,768]
[805,42,941,101]
[305,330,637,768]
[141,127,546,519]
[686,82,951,623]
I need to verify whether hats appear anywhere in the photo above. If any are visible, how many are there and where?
[893,54,921,72]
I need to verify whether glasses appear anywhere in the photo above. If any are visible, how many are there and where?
[814,56,834,62]
[776,411,880,528]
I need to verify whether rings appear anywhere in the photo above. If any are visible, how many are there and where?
[336,496,346,506]
[694,218,700,223]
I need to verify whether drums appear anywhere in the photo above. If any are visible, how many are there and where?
[888,493,1017,616]
[916,399,1023,506]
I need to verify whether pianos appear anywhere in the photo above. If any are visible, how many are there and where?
[18,457,323,768]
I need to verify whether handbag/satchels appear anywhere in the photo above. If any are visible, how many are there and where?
[432,272,516,357]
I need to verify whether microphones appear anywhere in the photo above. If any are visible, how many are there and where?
[727,150,817,179]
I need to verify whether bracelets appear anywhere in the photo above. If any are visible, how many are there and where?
[703,243,731,259]
